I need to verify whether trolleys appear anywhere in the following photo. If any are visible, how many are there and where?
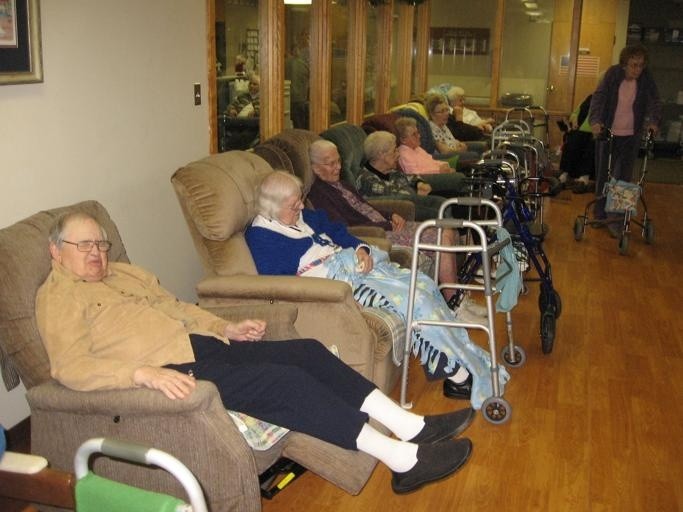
[572,127,658,255]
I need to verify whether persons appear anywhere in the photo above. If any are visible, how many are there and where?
[221,47,342,126]
[244,168,508,400]
[355,129,492,252]
[587,43,663,237]
[33,208,475,495]
[547,95,603,197]
[302,139,494,329]
[392,83,538,176]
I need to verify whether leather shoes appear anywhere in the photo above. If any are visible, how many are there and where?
[443,372,473,400]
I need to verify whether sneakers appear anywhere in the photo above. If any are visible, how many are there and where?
[397,406,475,444]
[591,215,607,228]
[459,295,488,317]
[453,305,488,328]
[389,437,472,494]
[491,268,497,280]
[572,178,594,194]
[609,220,622,240]
[474,270,485,285]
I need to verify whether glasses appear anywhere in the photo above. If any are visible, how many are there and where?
[433,108,449,114]
[61,239,112,252]
[318,157,342,167]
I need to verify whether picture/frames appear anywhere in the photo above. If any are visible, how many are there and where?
[0,0,45,86]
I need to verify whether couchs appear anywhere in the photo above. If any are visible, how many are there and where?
[253,100,518,282]
[171,150,434,397]
[0,200,391,512]
